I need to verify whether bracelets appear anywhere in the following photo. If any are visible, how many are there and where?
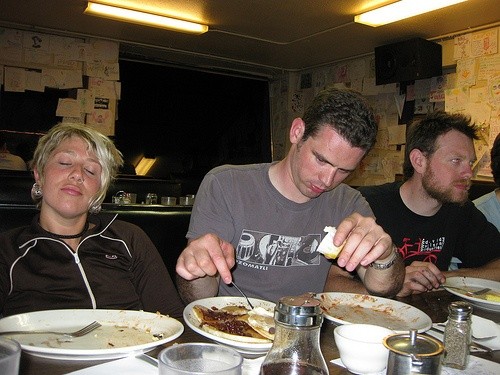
[368,244,399,271]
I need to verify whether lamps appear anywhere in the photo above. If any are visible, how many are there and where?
[135,156,157,176]
[82,2,209,36]
[354,0,467,28]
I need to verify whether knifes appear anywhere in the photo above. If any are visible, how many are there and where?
[430,327,493,353]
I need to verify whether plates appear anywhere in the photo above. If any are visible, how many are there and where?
[316,291,433,335]
[0,308,185,360]
[441,277,500,312]
[183,296,277,355]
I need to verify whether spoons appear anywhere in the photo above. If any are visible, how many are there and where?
[438,325,497,340]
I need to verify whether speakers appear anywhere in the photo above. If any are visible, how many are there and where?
[373,37,443,85]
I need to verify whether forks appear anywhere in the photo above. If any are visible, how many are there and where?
[0,321,102,339]
[437,286,491,296]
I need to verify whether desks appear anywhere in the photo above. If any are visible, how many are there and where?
[18,289,500,375]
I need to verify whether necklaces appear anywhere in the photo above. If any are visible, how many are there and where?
[47,220,89,240]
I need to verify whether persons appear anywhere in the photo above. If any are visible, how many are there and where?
[1,122,186,320]
[175,86,406,309]
[323,106,500,297]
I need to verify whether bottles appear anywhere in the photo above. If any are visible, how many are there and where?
[259,296,329,375]
[440,301,473,368]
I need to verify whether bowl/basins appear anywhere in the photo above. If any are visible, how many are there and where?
[111,192,195,206]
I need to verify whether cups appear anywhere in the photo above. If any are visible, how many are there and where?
[334,324,396,374]
[158,342,244,375]
[0,338,21,375]
[383,328,445,374]
